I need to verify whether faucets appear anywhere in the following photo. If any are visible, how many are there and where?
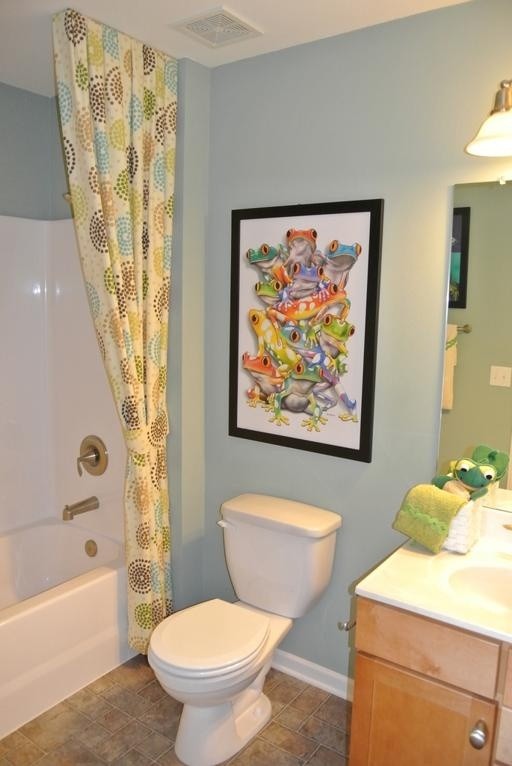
[62,496,99,520]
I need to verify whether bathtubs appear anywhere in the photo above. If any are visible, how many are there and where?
[0,518,142,739]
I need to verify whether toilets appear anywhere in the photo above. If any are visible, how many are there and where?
[147,493,343,765]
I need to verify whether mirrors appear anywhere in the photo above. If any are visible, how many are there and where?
[435,179,512,515]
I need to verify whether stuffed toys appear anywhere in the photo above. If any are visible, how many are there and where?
[468,445,509,482]
[431,455,498,502]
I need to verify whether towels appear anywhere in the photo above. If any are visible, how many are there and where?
[442,320,467,412]
[392,483,466,554]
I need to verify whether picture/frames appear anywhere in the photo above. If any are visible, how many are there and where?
[226,198,384,465]
[447,207,466,310]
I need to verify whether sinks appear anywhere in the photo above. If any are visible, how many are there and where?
[441,559,512,617]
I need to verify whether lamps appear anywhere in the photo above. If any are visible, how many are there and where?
[462,78,512,162]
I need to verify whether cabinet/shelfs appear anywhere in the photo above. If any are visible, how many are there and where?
[345,597,512,766]
